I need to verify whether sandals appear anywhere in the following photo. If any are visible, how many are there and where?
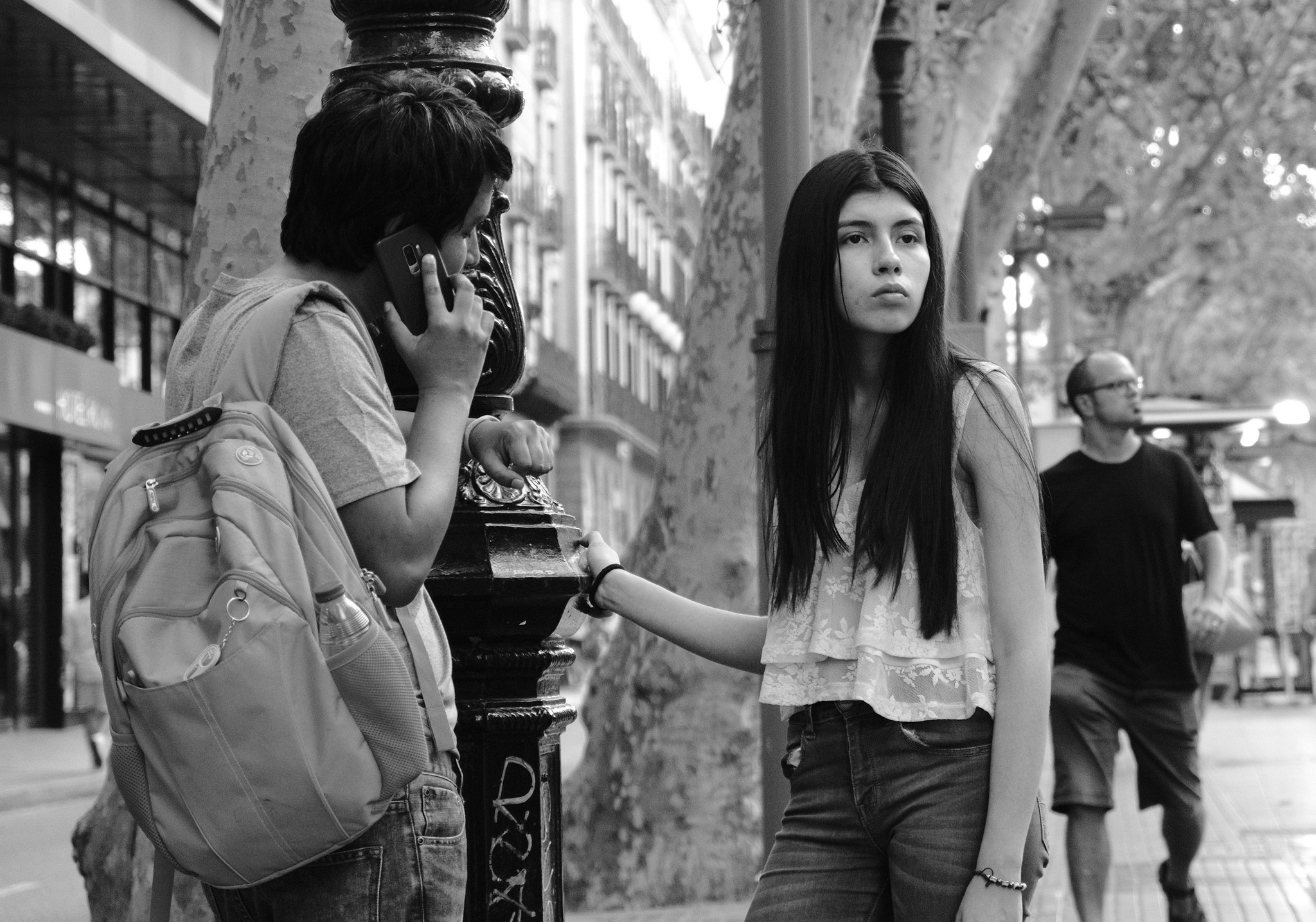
[1159,859,1206,922]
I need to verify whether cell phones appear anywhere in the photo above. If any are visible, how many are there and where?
[373,224,457,338]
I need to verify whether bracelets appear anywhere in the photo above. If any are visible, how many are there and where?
[465,414,500,458]
[974,868,1027,893]
[588,564,625,607]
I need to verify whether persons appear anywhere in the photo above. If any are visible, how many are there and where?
[578,151,1048,922]
[1032,350,1223,922]
[166,67,558,922]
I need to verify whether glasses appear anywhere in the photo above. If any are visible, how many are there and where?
[1089,375,1144,396]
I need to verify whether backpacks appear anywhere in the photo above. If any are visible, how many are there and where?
[81,277,452,891]
